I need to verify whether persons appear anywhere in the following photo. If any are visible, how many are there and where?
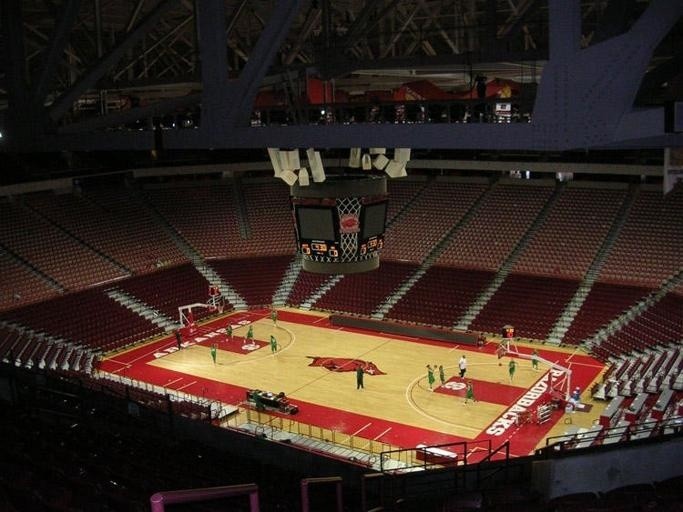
[357,366,365,389]
[171,300,278,362]
[253,392,265,411]
[427,328,580,421]
